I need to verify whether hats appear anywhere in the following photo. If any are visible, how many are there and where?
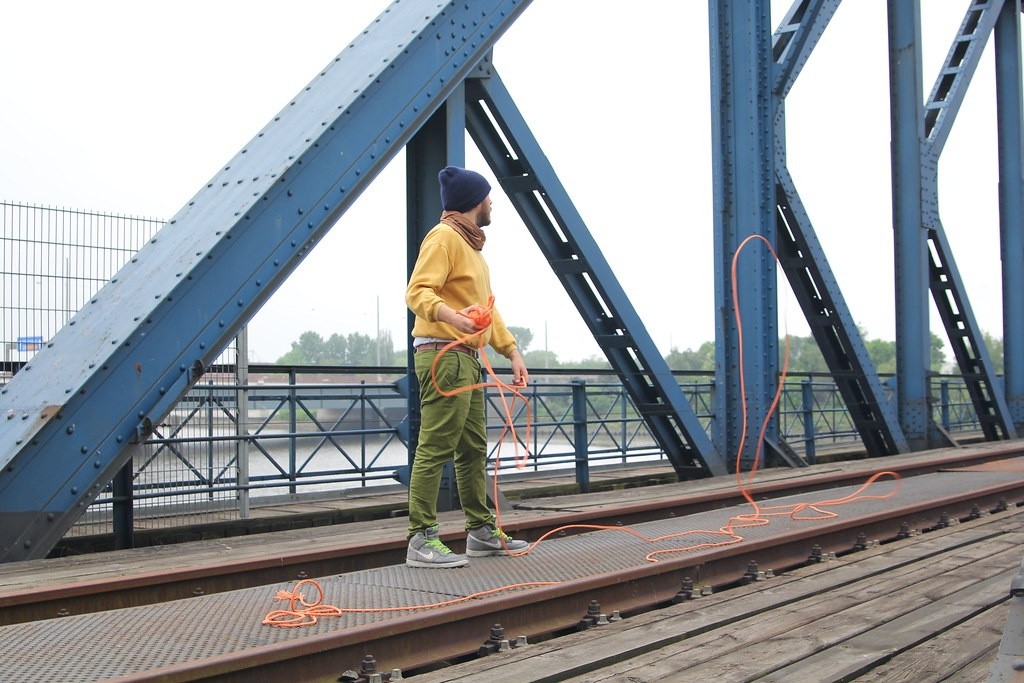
[438,166,492,214]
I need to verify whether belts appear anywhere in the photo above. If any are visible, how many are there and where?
[416,342,480,359]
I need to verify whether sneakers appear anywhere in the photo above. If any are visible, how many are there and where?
[406,524,469,568]
[466,524,530,557]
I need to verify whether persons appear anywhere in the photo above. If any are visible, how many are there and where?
[404,165,531,569]
